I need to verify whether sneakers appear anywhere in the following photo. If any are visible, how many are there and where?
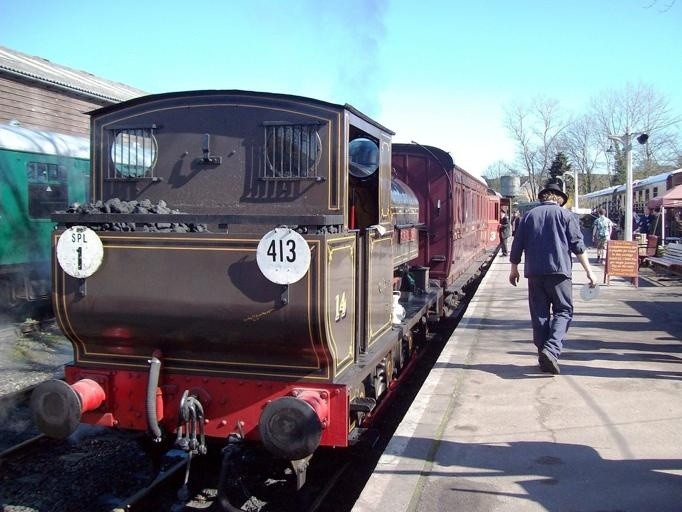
[595,259,604,263]
[540,348,561,375]
[500,252,507,256]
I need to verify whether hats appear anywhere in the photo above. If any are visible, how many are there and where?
[538,184,567,207]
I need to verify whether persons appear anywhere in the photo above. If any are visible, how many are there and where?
[511,210,516,222]
[617,205,662,242]
[510,210,519,238]
[496,208,512,258]
[509,183,599,376]
[592,208,613,266]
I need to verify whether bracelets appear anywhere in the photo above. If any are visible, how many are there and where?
[592,235,595,237]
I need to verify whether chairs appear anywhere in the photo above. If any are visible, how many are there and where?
[637,235,658,270]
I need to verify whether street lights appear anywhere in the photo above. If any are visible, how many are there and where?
[607,124,651,243]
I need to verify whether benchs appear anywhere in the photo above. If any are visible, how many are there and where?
[642,242,682,278]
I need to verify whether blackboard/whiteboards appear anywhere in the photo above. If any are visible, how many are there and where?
[607,240,639,278]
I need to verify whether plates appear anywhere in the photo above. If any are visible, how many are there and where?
[579,283,601,301]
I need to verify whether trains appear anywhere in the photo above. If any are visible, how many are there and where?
[26,89,510,497]
[577,168,682,244]
[1,119,156,310]
